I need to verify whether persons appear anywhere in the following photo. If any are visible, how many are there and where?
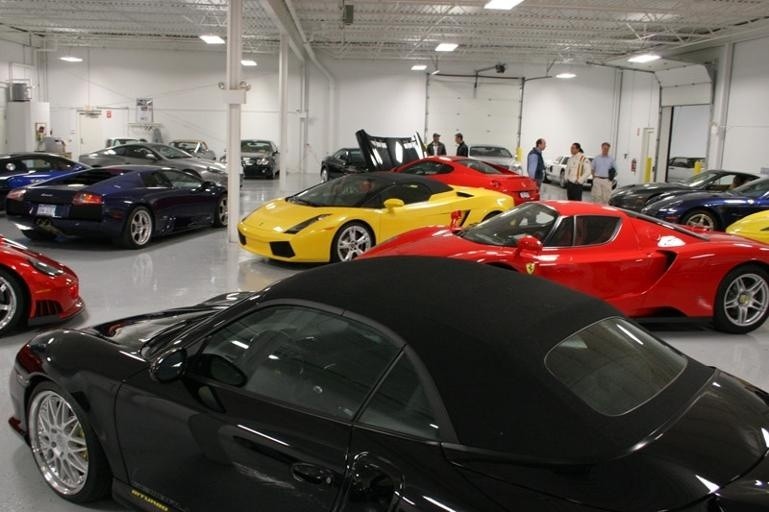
[36,126,44,141]
[453,133,469,157]
[590,142,619,204]
[564,142,592,201]
[427,133,447,156]
[526,138,547,193]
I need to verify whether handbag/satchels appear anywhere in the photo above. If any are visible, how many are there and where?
[609,168,616,180]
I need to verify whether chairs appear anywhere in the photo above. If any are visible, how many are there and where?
[555,218,610,247]
[151,174,166,187]
[358,179,373,193]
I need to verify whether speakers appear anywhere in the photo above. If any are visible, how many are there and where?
[344,5,353,23]
